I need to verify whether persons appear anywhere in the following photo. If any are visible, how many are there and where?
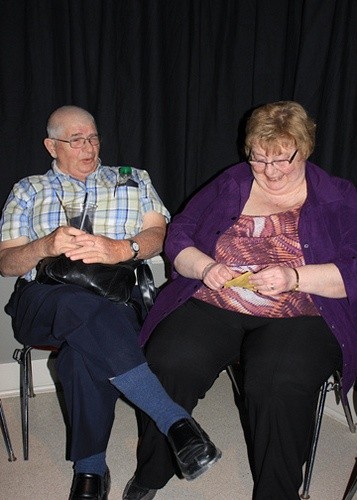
[0,107,222,500]
[122,100,357,500]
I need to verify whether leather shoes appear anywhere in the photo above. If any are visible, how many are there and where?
[167,416,222,481]
[68,465,111,500]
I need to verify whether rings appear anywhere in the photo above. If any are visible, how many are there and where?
[92,241,95,246]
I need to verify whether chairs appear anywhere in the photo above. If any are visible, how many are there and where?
[18,256,156,460]
[300,373,357,500]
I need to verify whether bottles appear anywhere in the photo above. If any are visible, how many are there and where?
[117,168,138,187]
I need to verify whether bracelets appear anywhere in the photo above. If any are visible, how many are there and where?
[291,268,299,292]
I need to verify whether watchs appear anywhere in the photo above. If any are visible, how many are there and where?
[126,239,139,260]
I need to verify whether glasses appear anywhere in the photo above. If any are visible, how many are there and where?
[51,135,103,148]
[247,149,298,169]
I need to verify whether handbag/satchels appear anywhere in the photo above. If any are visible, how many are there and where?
[35,252,155,325]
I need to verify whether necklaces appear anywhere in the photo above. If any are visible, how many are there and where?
[55,192,91,229]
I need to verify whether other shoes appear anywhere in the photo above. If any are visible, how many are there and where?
[122,475,157,500]
